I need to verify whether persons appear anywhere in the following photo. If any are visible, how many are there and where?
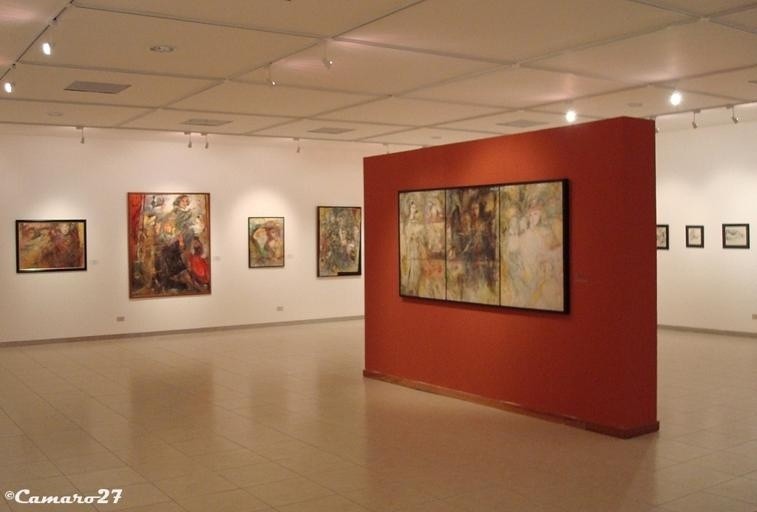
[505,183,558,309]
[155,196,208,291]
[401,201,429,297]
[447,190,495,295]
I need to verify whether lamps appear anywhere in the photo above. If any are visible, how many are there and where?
[650,111,660,130]
[726,104,741,124]
[689,108,702,128]
[41,22,59,58]
[322,38,334,68]
[3,63,17,94]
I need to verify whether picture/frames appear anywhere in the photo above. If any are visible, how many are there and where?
[247,217,285,267]
[316,206,362,279]
[722,224,749,248]
[398,178,570,316]
[127,191,212,299]
[686,226,704,247]
[656,224,669,249]
[15,219,87,274]
